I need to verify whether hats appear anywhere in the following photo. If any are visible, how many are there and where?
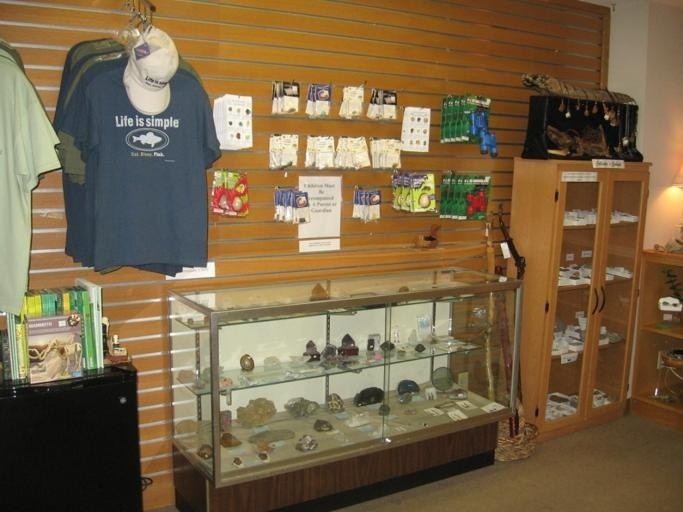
[123,25,179,117]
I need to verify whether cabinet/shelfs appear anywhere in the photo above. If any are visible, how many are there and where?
[633,248,683,428]
[165,267,524,511]
[500,157,651,433]
[0,368,143,511]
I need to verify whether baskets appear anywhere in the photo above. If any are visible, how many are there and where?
[495,421,539,463]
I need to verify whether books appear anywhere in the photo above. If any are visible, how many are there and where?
[0,276,106,388]
